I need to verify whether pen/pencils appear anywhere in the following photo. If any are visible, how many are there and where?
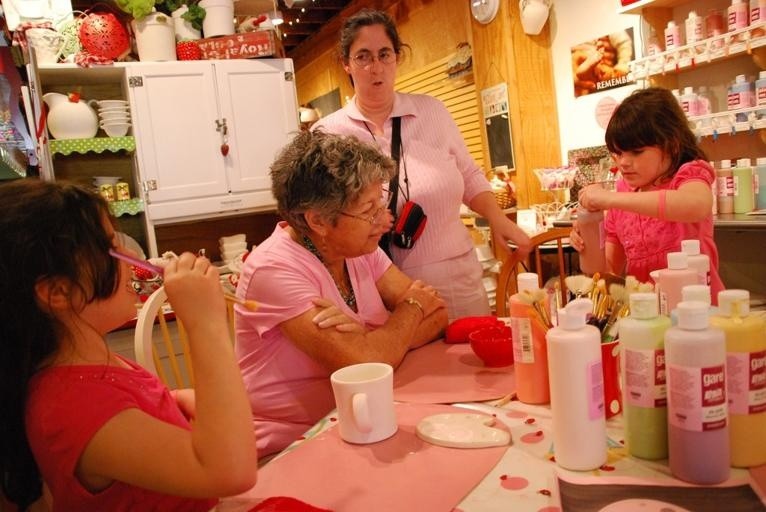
[533,272,628,343]
[492,390,517,408]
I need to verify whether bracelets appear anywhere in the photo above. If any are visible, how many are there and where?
[397,297,425,313]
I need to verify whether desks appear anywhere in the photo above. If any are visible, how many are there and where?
[200,338,764,512]
[505,234,577,277]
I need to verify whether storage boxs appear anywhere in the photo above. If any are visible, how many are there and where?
[197,30,283,59]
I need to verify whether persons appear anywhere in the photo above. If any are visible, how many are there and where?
[569,86,728,307]
[0,177,260,511]
[307,8,531,322]
[233,124,450,474]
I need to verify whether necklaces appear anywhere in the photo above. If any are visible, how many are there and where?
[308,240,358,307]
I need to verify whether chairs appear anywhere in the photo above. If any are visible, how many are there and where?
[133,262,240,394]
[493,229,585,320]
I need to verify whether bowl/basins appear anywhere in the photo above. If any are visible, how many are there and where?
[467,325,513,366]
[92,174,123,195]
[95,99,132,138]
[216,232,249,267]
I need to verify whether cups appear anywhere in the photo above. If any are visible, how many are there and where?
[24,28,68,66]
[596,336,622,420]
[330,360,400,448]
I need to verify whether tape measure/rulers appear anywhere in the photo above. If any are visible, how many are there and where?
[109,248,163,275]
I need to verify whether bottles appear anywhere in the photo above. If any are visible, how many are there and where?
[617,290,671,464]
[645,0,766,64]
[660,238,712,315]
[669,68,766,126]
[663,284,766,484]
[707,158,765,215]
[543,299,612,475]
[508,272,551,407]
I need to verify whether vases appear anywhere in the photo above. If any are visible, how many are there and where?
[172,6,201,40]
[127,13,177,61]
[199,0,235,38]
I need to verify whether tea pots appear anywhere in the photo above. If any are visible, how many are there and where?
[40,91,101,140]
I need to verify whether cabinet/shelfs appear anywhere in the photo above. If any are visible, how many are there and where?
[28,61,149,269]
[121,56,301,224]
[618,1,766,230]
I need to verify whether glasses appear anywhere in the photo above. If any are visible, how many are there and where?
[345,46,396,68]
[341,187,394,225]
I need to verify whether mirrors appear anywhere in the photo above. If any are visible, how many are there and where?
[484,112,515,171]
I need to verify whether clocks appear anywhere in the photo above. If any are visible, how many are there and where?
[468,0,499,26]
